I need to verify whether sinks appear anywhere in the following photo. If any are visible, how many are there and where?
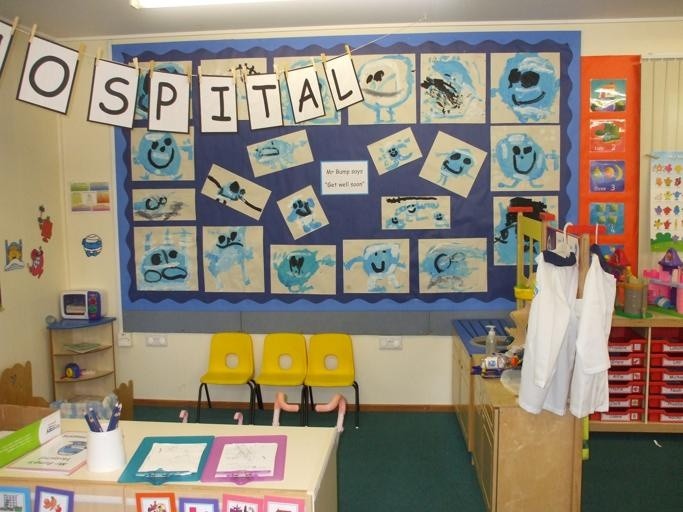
[469,335,511,348]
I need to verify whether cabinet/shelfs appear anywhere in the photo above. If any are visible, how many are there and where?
[45,318,118,403]
[450,316,583,512]
[586,315,683,437]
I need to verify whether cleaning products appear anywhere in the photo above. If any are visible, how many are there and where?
[486,325,496,354]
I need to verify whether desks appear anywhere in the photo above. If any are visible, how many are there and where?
[0,420,339,512]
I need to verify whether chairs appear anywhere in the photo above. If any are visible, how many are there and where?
[194,333,361,432]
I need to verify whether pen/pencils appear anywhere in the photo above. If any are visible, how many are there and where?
[83,402,122,432]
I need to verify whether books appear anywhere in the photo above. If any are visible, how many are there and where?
[4,430,93,476]
[62,343,102,353]
